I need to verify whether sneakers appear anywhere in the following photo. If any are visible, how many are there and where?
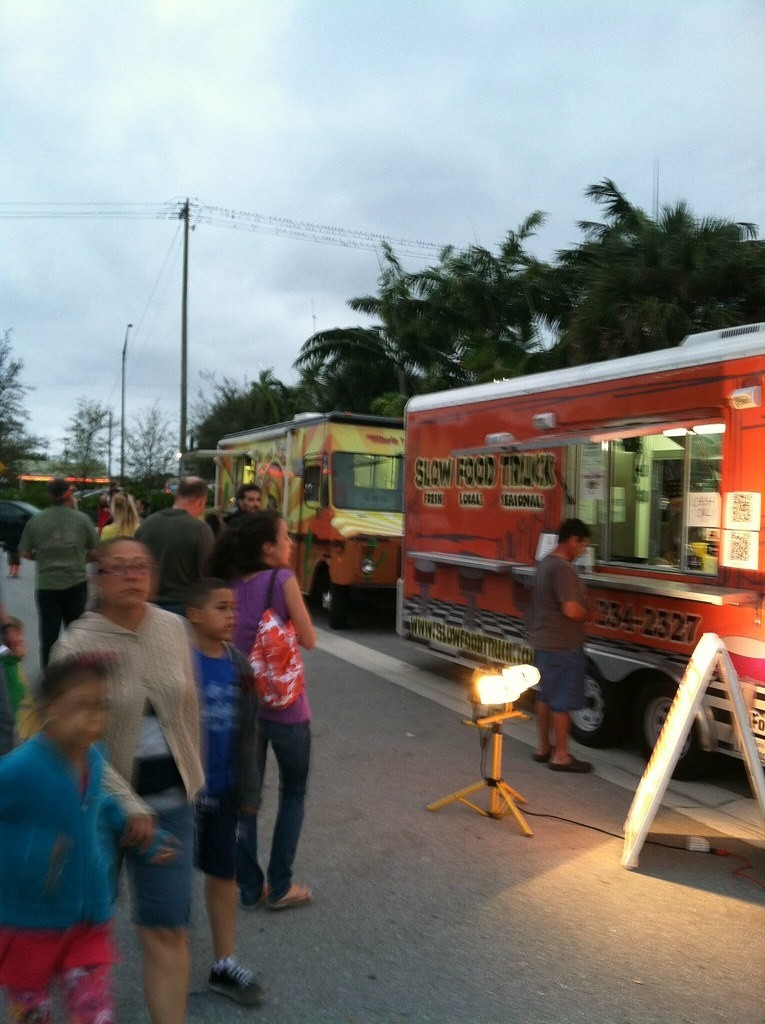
[208,959,264,1006]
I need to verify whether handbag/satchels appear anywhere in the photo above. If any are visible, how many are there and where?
[247,568,303,712]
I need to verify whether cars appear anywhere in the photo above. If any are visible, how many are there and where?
[165,479,180,495]
[0,500,41,546]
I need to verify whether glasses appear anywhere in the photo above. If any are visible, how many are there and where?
[97,562,150,575]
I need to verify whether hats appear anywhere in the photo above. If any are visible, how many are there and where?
[48,479,70,500]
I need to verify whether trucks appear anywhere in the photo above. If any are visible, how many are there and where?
[182,412,405,631]
[397,319,765,770]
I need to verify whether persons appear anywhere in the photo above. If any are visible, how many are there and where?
[528,519,604,773]
[0,477,316,1024]
[657,493,683,563]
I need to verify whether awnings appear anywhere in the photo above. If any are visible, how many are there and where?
[180,449,261,486]
[450,409,725,495]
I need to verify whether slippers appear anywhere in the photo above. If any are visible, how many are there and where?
[241,885,269,911]
[549,753,590,772]
[267,887,313,909]
[532,751,551,762]
